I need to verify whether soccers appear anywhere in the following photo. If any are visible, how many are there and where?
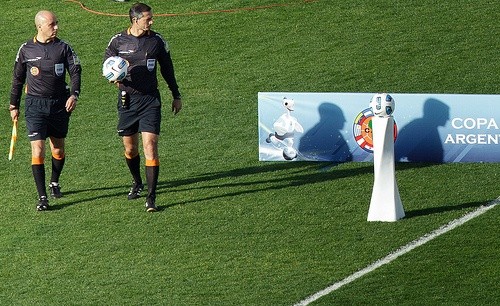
[102,56,131,84]
[368,92,395,117]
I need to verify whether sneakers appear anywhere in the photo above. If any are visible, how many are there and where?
[128,184,144,199]
[37,196,48,210]
[48,183,62,199]
[145,198,157,211]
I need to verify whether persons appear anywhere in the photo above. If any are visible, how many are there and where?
[9,9,83,214]
[100,1,183,213]
